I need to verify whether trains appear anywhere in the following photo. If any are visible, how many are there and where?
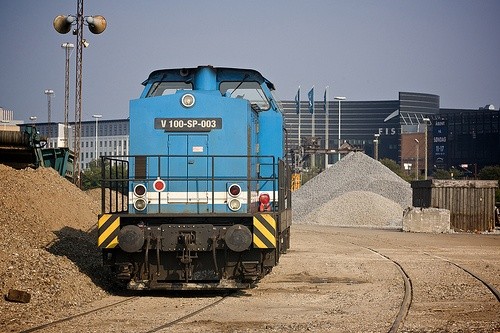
[99,66,292,291]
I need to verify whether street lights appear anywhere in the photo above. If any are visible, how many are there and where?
[92,115,102,166]
[335,96,346,161]
